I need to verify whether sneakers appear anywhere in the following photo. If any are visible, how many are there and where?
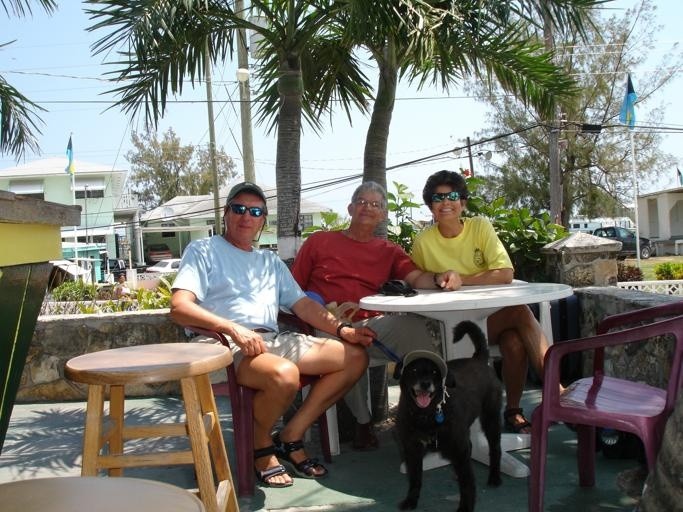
[352,415,380,451]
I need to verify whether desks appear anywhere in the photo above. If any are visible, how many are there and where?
[65,343,242,512]
[360,279,574,480]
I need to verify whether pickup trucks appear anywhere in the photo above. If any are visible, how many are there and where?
[590,227,653,259]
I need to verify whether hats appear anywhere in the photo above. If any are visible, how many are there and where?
[280,291,325,315]
[226,182,266,206]
[400,349,448,389]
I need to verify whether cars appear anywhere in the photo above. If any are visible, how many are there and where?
[143,243,172,266]
[146,258,181,275]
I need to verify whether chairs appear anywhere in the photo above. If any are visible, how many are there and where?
[530,300,683,512]
[489,279,555,359]
[301,352,391,455]
[187,326,332,496]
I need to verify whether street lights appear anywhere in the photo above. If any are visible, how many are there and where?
[84,183,89,272]
[628,130,642,270]
[453,136,493,216]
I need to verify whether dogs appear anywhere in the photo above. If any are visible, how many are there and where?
[392,320,503,512]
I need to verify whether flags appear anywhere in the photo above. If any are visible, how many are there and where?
[678,168,683,185]
[65,136,75,176]
[618,72,638,129]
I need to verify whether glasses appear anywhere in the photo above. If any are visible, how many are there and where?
[224,203,263,218]
[352,199,381,210]
[431,191,461,203]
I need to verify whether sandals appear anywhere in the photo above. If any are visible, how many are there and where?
[251,445,294,488]
[272,430,329,479]
[503,405,532,434]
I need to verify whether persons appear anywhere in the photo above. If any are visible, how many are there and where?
[169,181,378,488]
[289,179,463,452]
[112,273,131,299]
[409,170,585,436]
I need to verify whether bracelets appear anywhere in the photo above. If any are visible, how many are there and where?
[433,273,445,290]
[337,321,352,339]
